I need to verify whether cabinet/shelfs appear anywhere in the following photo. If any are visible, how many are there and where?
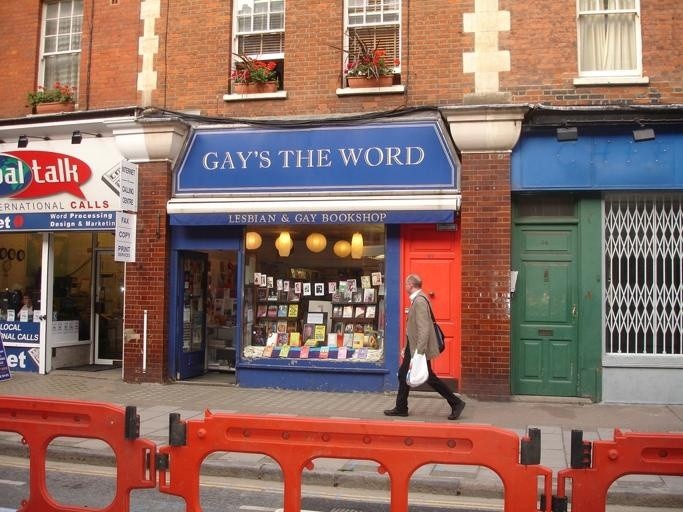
[203,278,380,372]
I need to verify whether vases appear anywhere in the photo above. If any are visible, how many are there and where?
[347,75,396,89]
[35,99,75,115]
[232,82,280,95]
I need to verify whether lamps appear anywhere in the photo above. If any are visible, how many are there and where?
[245,221,364,260]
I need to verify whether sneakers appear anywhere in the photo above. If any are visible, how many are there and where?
[448,400,466,420]
[384,407,409,416]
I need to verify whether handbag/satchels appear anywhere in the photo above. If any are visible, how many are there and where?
[406,348,429,388]
[434,324,446,353]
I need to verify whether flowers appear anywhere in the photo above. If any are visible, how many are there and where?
[26,81,78,108]
[326,24,401,87]
[226,47,278,87]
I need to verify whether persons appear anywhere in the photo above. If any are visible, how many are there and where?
[384,274,466,420]
[17,293,34,318]
